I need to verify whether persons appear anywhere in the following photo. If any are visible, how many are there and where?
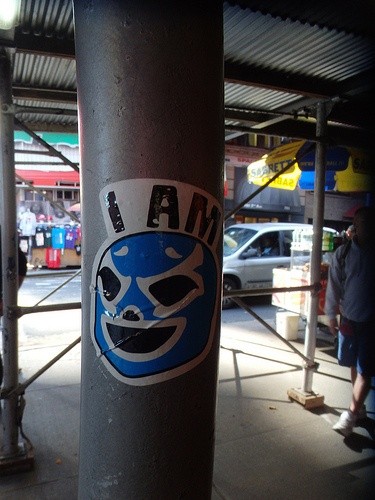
[323,206,375,437]
[261,234,280,256]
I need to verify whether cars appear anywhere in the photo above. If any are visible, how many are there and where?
[221,223,341,310]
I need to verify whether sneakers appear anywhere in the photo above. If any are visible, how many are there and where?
[356,406,367,420]
[333,410,357,437]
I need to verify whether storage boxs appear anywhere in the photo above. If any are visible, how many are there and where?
[290,249,333,278]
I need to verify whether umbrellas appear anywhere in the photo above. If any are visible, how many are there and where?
[247,140,373,192]
